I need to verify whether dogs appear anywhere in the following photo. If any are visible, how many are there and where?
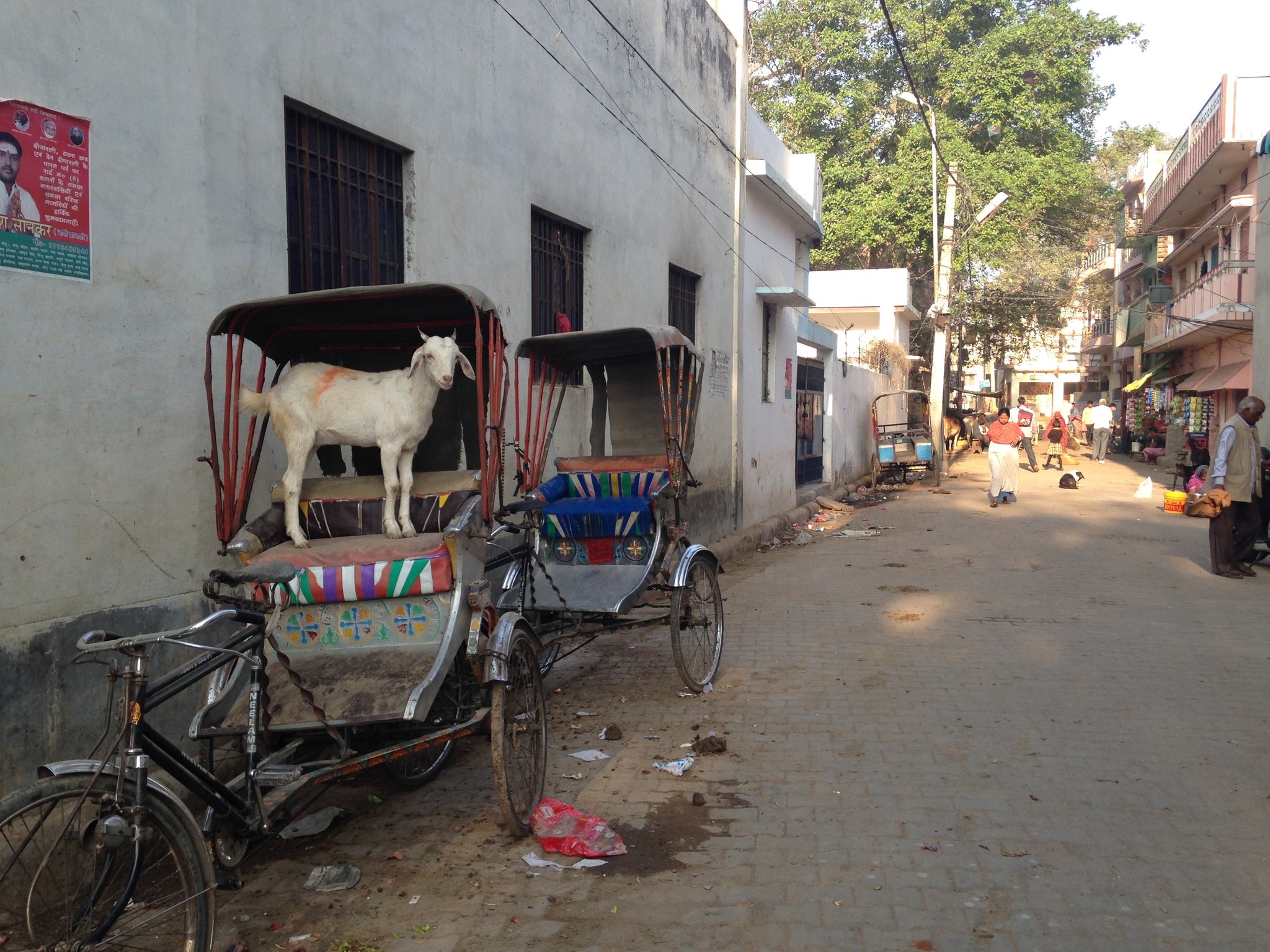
[1059,470,1085,490]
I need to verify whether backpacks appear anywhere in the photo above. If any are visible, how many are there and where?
[1017,408,1033,427]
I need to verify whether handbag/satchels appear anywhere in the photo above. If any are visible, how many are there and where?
[1133,476,1153,498]
[1184,489,1231,518]
[1081,421,1087,432]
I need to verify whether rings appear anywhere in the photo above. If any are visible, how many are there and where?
[12,210,17,213]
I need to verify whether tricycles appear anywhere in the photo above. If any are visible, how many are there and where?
[0,283,547,952]
[366,324,728,786]
[871,389,941,487]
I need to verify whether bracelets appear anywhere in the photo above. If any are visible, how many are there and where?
[982,430,986,432]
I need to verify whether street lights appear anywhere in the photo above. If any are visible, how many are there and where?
[925,161,1010,481]
[898,92,939,305]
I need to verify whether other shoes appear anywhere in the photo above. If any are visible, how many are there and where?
[1216,567,1243,579]
[1057,467,1063,471]
[1243,549,1268,564]
[1105,450,1110,455]
[1255,536,1268,544]
[1032,467,1039,472]
[1099,460,1104,461]
[1142,460,1149,463]
[1042,464,1048,470]
[1090,458,1096,461]
[1234,563,1257,577]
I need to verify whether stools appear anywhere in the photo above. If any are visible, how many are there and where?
[972,438,983,454]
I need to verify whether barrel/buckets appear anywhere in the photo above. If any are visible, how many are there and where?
[1163,491,1188,514]
[878,444,894,462]
[916,443,932,460]
[1132,442,1140,452]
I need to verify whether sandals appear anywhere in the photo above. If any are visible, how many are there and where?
[1002,500,1010,504]
[990,501,998,507]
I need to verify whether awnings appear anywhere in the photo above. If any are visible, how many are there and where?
[1122,350,1248,394]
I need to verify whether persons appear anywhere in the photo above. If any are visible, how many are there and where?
[1042,420,1064,471]
[69,128,84,146]
[1186,464,1210,498]
[972,412,991,446]
[1069,402,1083,441]
[1042,411,1072,455]
[1143,428,1165,462]
[1081,401,1094,449]
[0,131,40,238]
[1209,394,1267,578]
[1090,399,1113,465]
[977,408,1024,507]
[1003,397,1039,472]
[41,120,56,139]
[1105,403,1120,451]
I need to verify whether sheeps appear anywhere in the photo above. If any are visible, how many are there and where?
[232,324,476,548]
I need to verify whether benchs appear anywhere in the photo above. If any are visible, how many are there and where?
[225,479,479,608]
[527,454,687,539]
[878,429,931,462]
